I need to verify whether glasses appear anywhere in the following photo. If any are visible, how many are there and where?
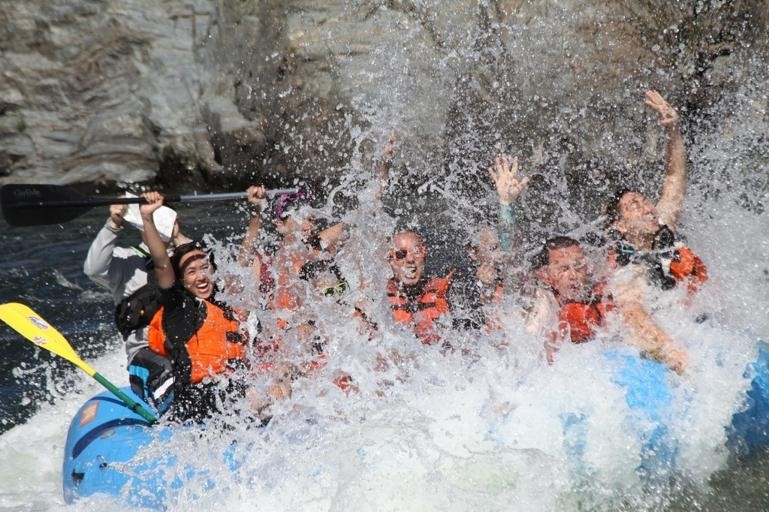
[320,279,348,298]
[389,244,421,262]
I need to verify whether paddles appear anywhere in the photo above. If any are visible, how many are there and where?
[0,303,158,424]
[1,183,298,227]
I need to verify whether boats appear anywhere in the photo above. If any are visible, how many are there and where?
[60,317,769,512]
